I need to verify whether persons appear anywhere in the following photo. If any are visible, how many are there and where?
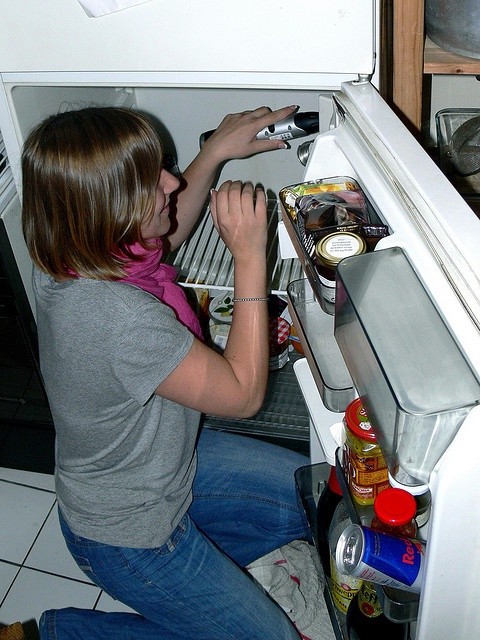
[0,100,311,640]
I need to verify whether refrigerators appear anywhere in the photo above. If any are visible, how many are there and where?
[1,2,480,636]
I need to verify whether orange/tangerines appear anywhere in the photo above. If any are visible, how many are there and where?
[291,340,302,353]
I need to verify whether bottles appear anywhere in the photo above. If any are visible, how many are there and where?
[346,580,410,640]
[329,497,373,616]
[314,464,345,582]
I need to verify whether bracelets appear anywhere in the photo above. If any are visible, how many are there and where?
[232,298,269,302]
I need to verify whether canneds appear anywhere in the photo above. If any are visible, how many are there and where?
[309,231,365,306]
[372,487,421,539]
[265,318,290,372]
[342,396,388,507]
[334,523,424,598]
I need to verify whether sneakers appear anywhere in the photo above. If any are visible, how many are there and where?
[0,618,40,640]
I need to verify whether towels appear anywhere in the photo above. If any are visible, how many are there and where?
[245,539,338,639]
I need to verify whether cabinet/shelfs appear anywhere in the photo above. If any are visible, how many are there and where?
[392,1,480,202]
